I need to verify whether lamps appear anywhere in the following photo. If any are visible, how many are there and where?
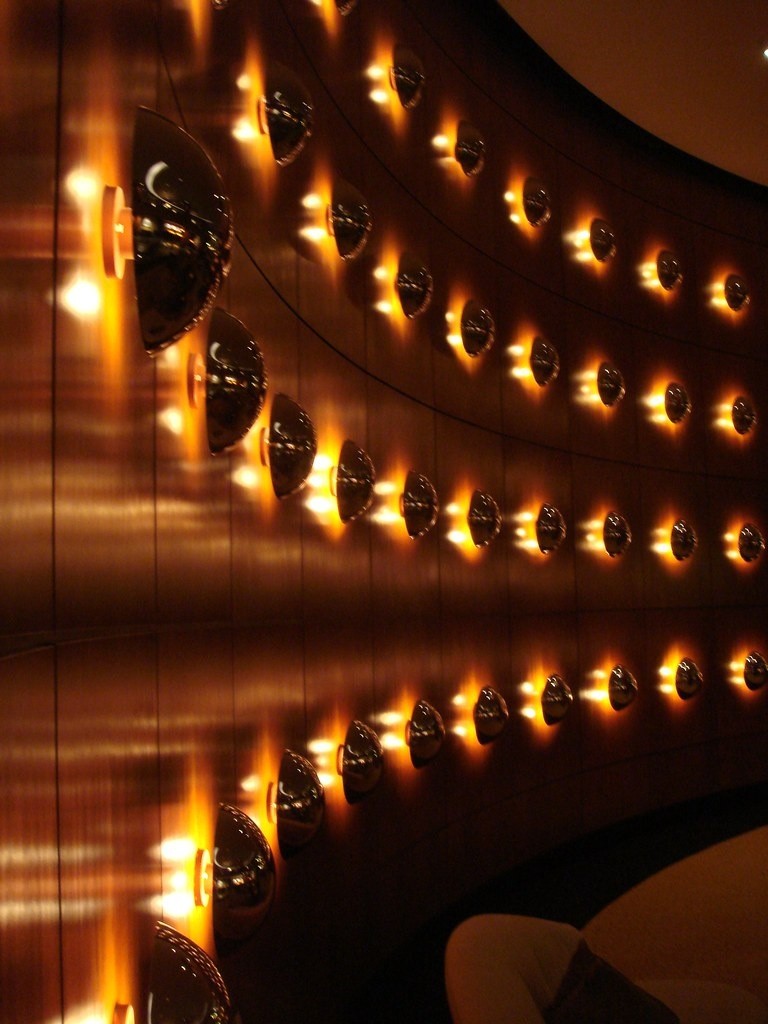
[471,685,512,748]
[522,170,768,724]
[102,102,235,357]
[330,439,377,523]
[256,63,315,167]
[405,698,447,773]
[111,921,241,1024]
[185,304,267,458]
[468,487,503,549]
[388,42,429,112]
[326,178,373,263]
[259,391,319,501]
[454,117,485,180]
[458,298,496,360]
[394,248,434,323]
[265,749,326,864]
[399,469,439,541]
[336,717,387,807]
[193,803,275,956]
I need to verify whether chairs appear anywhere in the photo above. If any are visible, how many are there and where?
[443,913,580,1024]
